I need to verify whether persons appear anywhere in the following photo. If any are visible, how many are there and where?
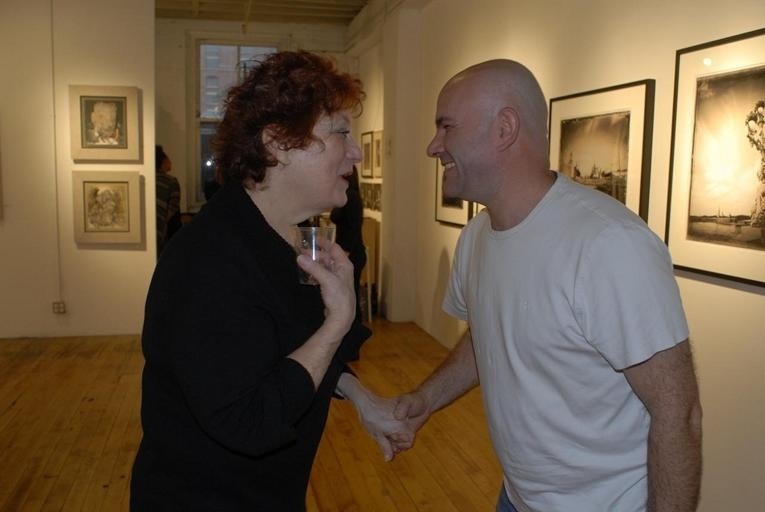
[156,145,181,260]
[131,51,416,512]
[330,165,367,322]
[390,60,702,511]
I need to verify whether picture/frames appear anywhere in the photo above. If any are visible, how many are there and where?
[549,78,657,229]
[358,131,383,224]
[68,83,143,246]
[434,160,476,227]
[666,28,763,287]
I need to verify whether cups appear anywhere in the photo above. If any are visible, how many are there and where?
[290,226,337,286]
